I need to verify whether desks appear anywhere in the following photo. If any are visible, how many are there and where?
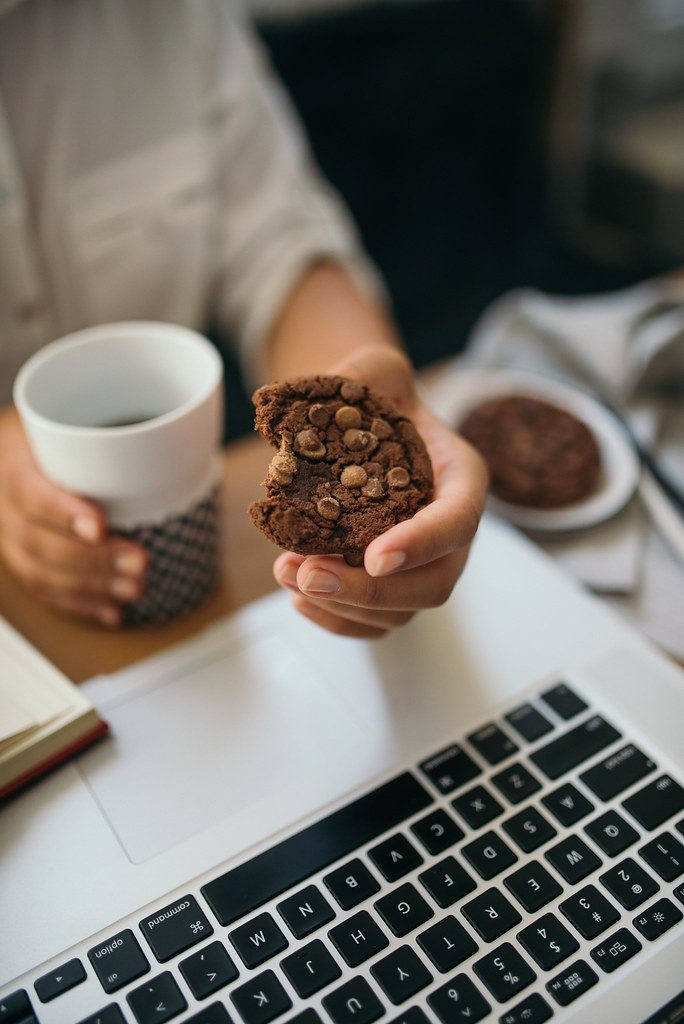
[0,271,684,670]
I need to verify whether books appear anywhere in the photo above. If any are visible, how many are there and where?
[0,616,109,814]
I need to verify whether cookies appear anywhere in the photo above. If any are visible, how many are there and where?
[247,375,432,565]
[456,395,600,507]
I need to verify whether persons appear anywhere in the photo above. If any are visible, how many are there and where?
[0,0,488,639]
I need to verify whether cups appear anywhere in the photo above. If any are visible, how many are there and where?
[12,319,224,628]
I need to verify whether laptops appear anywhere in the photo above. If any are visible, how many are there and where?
[1,510,684,1024]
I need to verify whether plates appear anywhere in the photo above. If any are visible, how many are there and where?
[434,365,641,532]
